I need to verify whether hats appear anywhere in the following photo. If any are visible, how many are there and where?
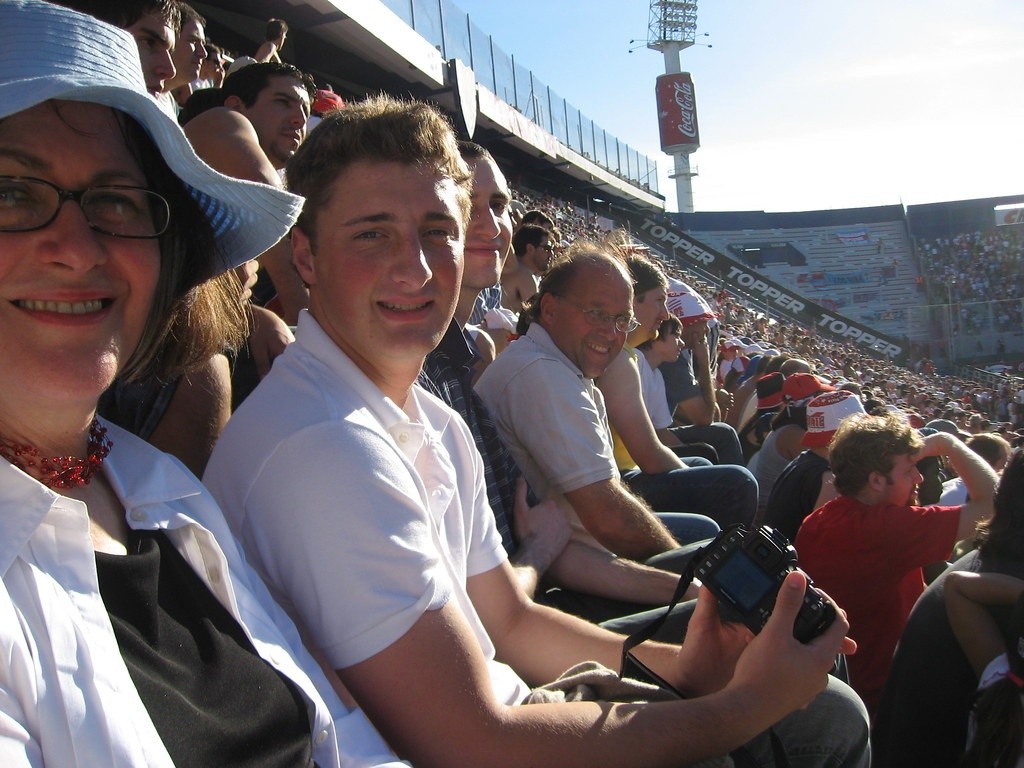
[510,200,526,213]
[785,373,837,400]
[662,292,718,327]
[724,338,779,355]
[800,390,870,447]
[747,355,763,371]
[226,55,259,77]
[926,419,971,438]
[909,414,925,428]
[0,0,306,279]
[756,372,787,409]
[731,356,751,373]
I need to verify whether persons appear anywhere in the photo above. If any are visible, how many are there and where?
[53,0,1024,768]
[0,0,412,768]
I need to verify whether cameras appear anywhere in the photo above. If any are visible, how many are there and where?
[691,521,836,644]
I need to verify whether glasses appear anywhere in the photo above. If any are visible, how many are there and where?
[539,241,554,250]
[0,175,170,239]
[549,292,641,333]
[209,58,219,64]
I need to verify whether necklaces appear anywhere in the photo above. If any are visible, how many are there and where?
[0,420,113,490]
